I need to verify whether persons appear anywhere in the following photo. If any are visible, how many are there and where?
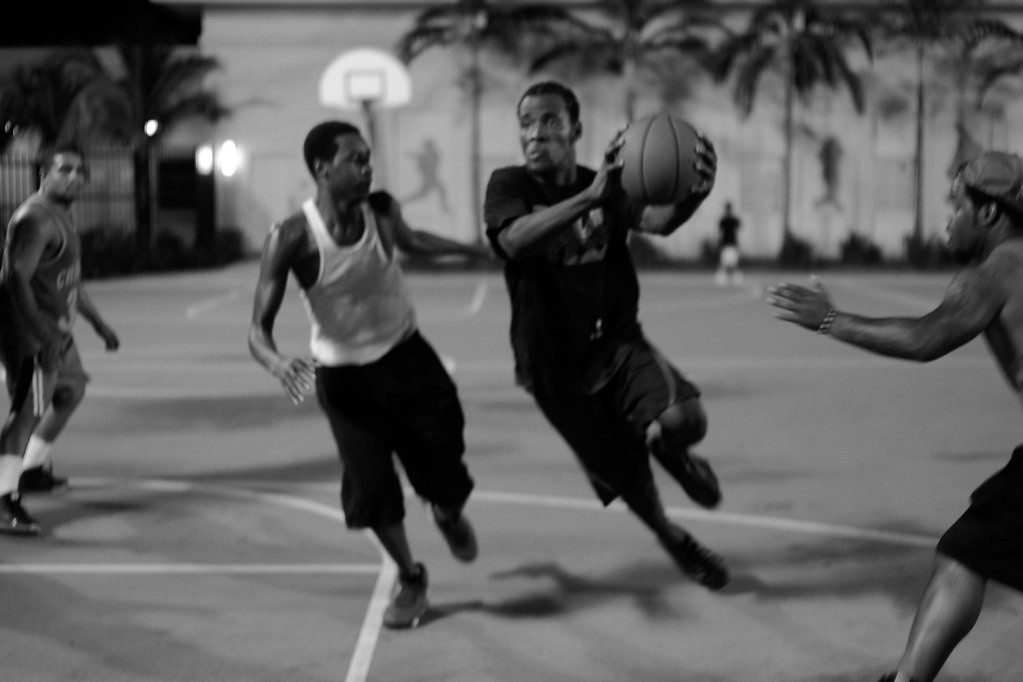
[713,203,744,282]
[766,148,1023,682]
[0,138,121,534]
[247,122,490,630]
[484,81,734,592]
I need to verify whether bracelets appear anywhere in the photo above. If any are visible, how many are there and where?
[819,306,837,334]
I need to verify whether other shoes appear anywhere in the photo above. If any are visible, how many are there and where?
[18,464,68,492]
[383,562,429,629]
[661,531,729,590]
[649,439,720,509]
[434,511,478,563]
[0,492,43,535]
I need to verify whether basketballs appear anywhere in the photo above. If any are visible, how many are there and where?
[611,114,702,207]
